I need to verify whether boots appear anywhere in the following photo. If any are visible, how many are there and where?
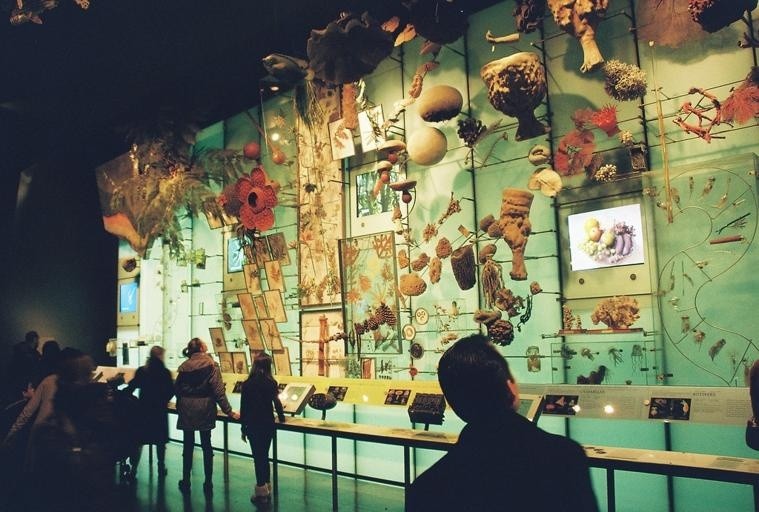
[250,481,271,511]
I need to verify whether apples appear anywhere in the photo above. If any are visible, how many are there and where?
[589,227,602,241]
[601,231,615,246]
[585,218,599,232]
[244,142,261,158]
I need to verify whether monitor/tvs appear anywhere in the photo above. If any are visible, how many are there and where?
[514,392,545,425]
[93,371,103,380]
[275,383,316,416]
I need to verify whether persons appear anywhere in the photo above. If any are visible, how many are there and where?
[0,331,93,512]
[239,352,285,507]
[404,334,601,512]
[174,338,239,493]
[120,346,173,480]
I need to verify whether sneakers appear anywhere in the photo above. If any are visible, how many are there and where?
[178,479,191,496]
[204,482,213,499]
